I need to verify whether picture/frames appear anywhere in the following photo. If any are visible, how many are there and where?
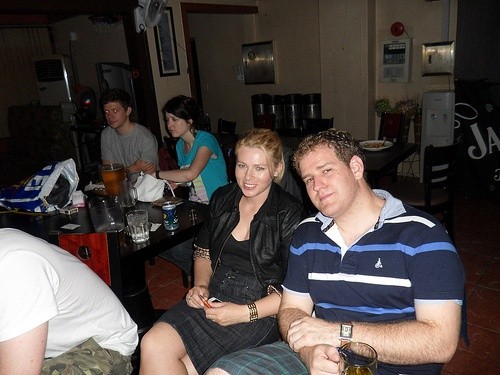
[421,40,455,76]
[153,6,180,77]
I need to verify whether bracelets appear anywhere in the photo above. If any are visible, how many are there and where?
[247,302,258,322]
[156,171,161,179]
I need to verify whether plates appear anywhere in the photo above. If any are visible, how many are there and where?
[359,140,394,151]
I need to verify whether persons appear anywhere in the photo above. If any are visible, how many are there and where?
[203,127,466,375]
[151,95,230,277]
[139,129,303,375]
[101,89,159,175]
[0,228,139,375]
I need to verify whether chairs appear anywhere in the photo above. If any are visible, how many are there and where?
[381,139,460,249]
[379,112,406,183]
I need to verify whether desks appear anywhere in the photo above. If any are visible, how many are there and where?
[212,132,419,220]
[0,190,208,375]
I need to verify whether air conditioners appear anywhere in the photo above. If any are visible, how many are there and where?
[34,56,89,169]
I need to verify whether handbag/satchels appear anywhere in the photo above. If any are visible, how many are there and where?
[89,202,126,233]
[131,171,175,202]
[0,158,80,213]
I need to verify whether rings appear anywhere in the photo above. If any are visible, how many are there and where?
[187,298,190,301]
[211,314,215,320]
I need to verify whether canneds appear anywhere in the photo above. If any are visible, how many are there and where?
[162,201,179,230]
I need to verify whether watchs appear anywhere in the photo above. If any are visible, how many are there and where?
[340,321,353,350]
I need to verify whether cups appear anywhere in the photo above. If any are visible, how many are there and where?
[188,203,200,220]
[338,341,378,375]
[101,162,138,207]
[88,193,125,234]
[125,209,150,243]
